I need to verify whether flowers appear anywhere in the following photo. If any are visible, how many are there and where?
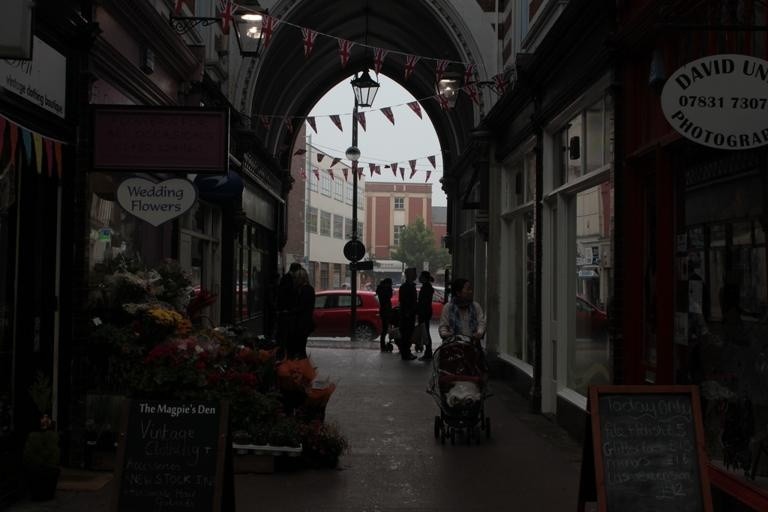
[83,253,225,394]
[226,326,345,459]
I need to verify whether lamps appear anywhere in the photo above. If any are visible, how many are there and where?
[167,8,270,57]
[349,1,380,107]
[433,74,506,114]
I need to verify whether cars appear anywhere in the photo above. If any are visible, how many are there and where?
[390,287,444,322]
[311,289,381,341]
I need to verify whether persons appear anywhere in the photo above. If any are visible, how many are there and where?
[279,262,315,359]
[416,271,435,360]
[399,268,416,361]
[449,379,478,404]
[438,280,486,341]
[376,278,393,351]
[341,282,349,306]
[364,282,373,292]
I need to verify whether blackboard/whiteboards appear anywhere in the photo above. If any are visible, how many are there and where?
[588,385,715,512]
[108,398,231,512]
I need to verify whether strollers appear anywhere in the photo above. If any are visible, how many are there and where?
[385,306,424,353]
[424,333,491,445]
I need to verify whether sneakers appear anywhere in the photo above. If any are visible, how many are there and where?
[401,353,433,360]
